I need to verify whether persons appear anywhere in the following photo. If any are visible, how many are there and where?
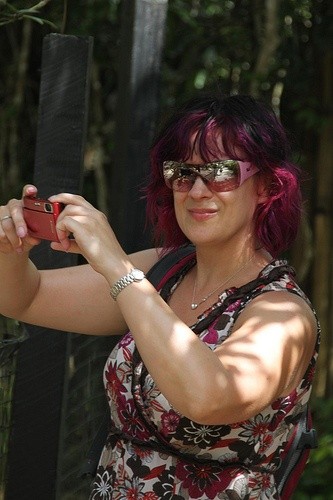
[0,96,321,500]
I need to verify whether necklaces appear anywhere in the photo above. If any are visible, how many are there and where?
[191,251,260,310]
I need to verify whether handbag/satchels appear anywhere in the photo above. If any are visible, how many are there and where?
[275,403,318,500]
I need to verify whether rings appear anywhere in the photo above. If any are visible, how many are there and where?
[0,216,12,223]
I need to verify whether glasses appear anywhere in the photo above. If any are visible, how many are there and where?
[162,158,261,192]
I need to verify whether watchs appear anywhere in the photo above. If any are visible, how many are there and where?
[110,268,146,301]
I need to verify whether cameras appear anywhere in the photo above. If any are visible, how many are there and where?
[22,195,64,242]
